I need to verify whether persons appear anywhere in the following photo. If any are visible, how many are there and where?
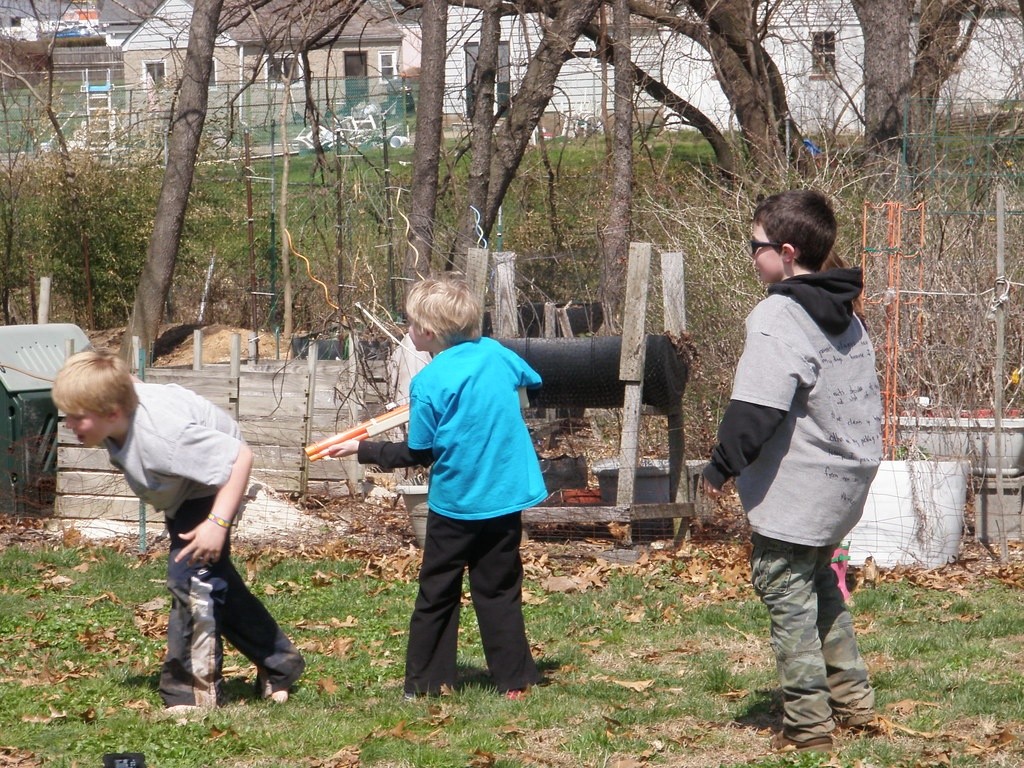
[703,190,883,752]
[330,271,546,699]
[54,347,304,707]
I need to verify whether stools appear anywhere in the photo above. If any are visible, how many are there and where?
[594,459,706,530]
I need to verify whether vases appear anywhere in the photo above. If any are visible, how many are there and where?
[399,485,430,550]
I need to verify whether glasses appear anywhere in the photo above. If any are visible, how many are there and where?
[750,240,795,255]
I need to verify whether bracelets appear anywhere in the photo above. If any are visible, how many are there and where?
[208,513,231,529]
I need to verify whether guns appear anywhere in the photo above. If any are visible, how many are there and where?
[304,384,533,461]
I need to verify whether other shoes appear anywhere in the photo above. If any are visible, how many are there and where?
[506,689,532,702]
[842,714,875,727]
[403,693,420,701]
[770,728,833,754]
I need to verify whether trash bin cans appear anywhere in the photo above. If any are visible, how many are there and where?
[0,323,105,515]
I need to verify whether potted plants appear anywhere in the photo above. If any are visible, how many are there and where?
[840,443,970,572]
